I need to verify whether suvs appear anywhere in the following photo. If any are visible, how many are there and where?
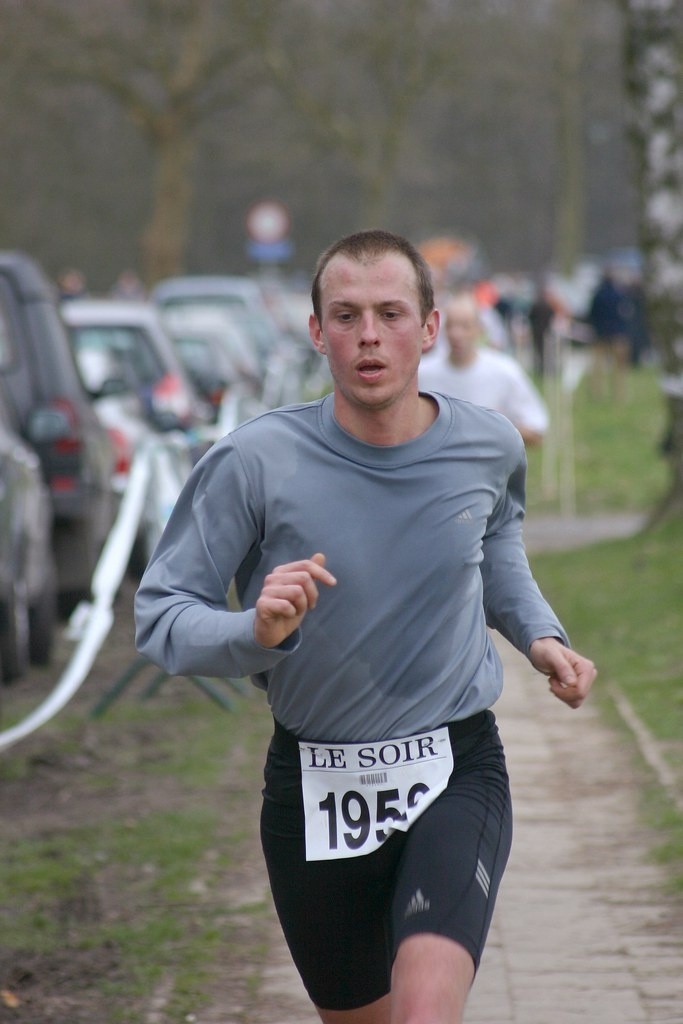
[0,248,137,616]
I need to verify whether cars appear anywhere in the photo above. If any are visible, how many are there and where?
[55,264,332,587]
[0,359,73,686]
